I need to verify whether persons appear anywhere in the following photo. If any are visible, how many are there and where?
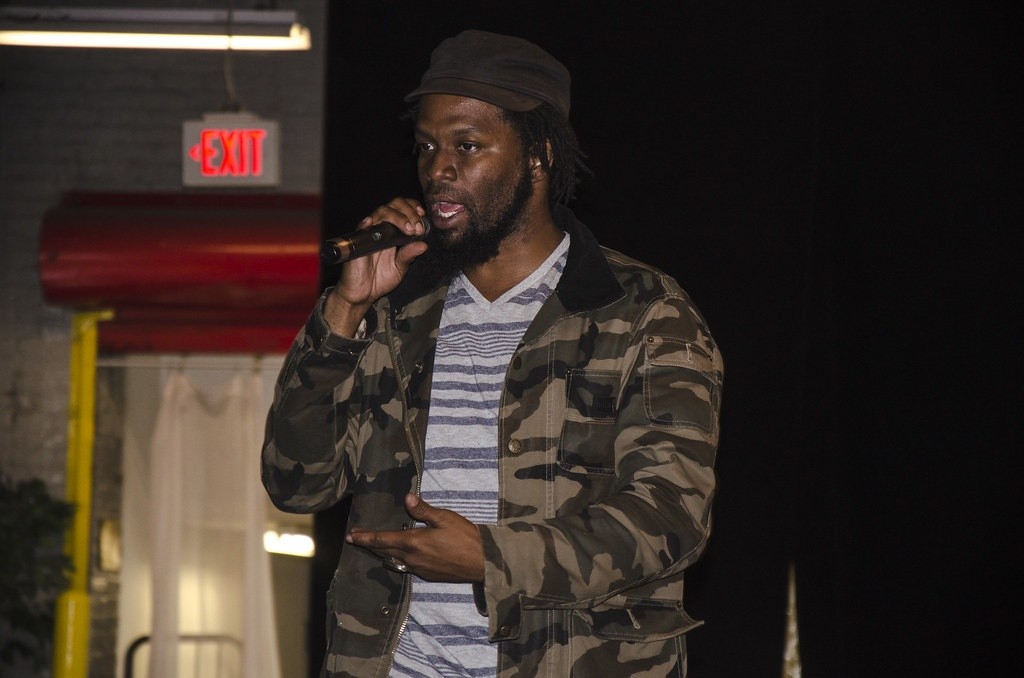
[261,29,724,678]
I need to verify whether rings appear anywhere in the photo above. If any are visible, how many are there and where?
[382,558,408,574]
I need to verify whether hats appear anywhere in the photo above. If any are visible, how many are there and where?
[403,29,572,128]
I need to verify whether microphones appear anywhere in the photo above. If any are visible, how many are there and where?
[321,215,432,265]
